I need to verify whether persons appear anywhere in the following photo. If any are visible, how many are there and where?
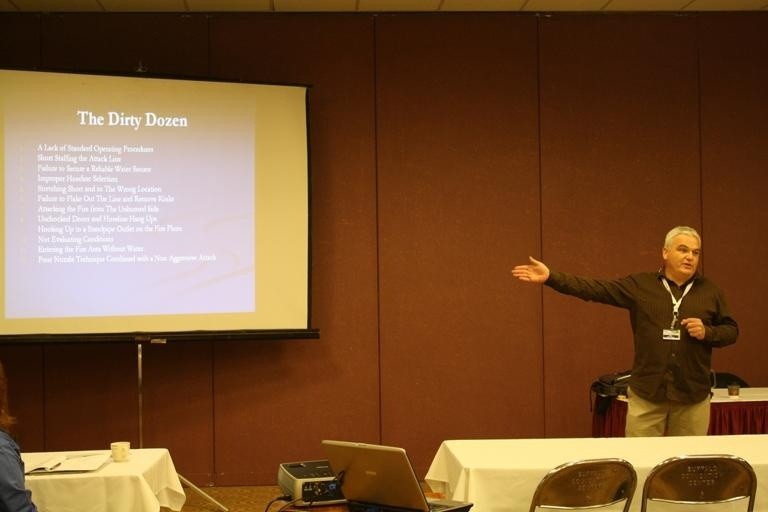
[1,363,38,512]
[511,225,739,437]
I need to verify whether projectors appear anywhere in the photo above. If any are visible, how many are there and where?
[278,459,347,507]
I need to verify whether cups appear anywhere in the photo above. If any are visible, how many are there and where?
[109,441,131,463]
[728,381,740,396]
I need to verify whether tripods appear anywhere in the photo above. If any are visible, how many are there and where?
[137,343,230,512]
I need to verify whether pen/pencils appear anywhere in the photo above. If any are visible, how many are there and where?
[50,462,61,470]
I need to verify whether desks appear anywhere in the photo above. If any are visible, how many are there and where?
[19,447,171,510]
[598,385,767,435]
[440,435,768,512]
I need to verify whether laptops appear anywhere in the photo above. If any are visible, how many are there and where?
[322,440,473,512]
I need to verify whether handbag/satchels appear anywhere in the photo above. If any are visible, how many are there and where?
[591,370,632,397]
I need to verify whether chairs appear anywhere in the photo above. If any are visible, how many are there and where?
[529,457,756,512]
